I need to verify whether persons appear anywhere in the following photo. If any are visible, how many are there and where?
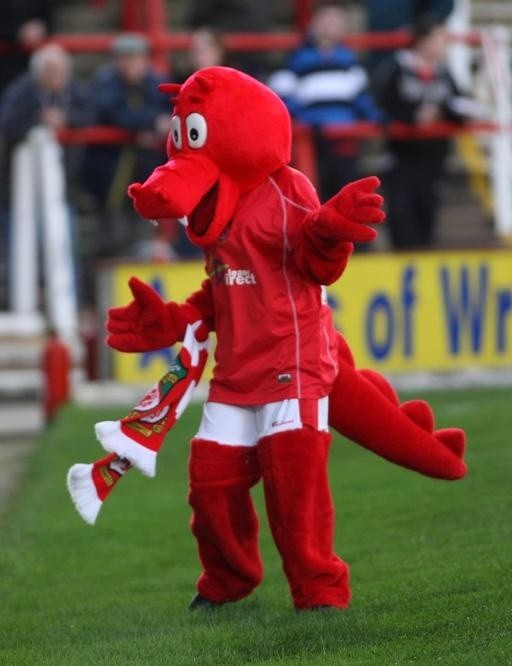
[42,329,71,424]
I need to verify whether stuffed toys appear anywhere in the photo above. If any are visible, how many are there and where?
[66,66,467,612]
